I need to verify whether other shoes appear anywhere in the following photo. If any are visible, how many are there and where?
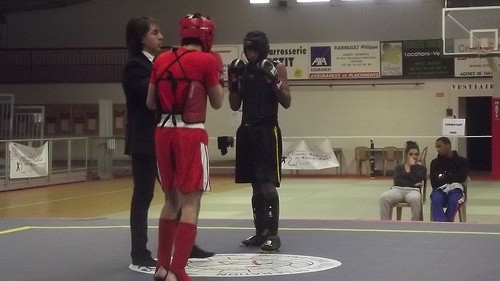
[188,245,215,262]
[154,267,193,281]
[131,255,158,270]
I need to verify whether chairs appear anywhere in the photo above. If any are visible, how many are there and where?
[430,175,471,222]
[382,147,398,176]
[390,185,424,220]
[416,147,428,168]
[355,146,372,177]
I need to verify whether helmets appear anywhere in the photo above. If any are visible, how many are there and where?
[242,30,270,63]
[180,14,215,51]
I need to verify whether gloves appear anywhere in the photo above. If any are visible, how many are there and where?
[227,58,245,90]
[258,59,283,91]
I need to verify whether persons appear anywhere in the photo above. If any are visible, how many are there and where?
[226,30,291,254]
[377,140,427,221]
[117,15,216,274]
[145,12,225,281]
[430,136,468,221]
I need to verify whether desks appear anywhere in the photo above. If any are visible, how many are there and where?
[288,147,342,175]
[362,148,404,177]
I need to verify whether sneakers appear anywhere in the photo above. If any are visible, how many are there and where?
[240,235,265,247]
[259,237,282,253]
